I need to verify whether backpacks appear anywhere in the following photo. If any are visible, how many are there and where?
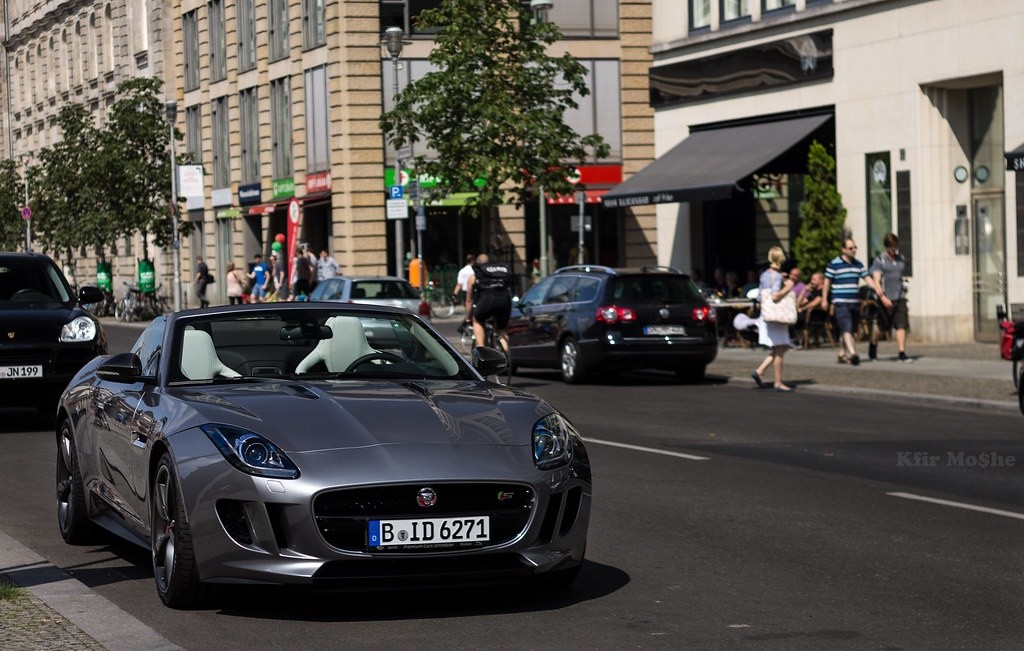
[473,262,512,292]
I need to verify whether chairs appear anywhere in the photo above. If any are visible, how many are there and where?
[180,329,242,379]
[803,301,891,350]
[376,288,402,297]
[295,315,393,373]
[354,288,366,298]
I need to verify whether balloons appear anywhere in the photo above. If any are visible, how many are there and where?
[275,234,285,242]
[271,242,281,251]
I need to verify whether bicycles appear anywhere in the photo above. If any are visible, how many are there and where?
[424,284,455,318]
[71,281,166,322]
[460,315,511,388]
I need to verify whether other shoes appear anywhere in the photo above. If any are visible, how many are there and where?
[837,354,852,363]
[774,384,794,393]
[869,342,878,360]
[849,354,860,365]
[751,371,765,388]
[898,354,912,364]
[206,302,210,308]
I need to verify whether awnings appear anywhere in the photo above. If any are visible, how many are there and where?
[599,110,832,212]
[541,186,613,206]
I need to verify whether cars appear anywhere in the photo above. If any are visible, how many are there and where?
[0,252,108,415]
[307,275,432,361]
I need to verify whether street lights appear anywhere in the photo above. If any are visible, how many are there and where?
[23,167,30,254]
[384,24,407,297]
[163,99,181,311]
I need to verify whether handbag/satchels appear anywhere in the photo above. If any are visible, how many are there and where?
[293,273,299,284]
[761,274,798,325]
[206,274,214,284]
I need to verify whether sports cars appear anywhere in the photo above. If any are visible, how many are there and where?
[54,301,592,609]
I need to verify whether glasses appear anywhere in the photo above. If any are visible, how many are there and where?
[845,246,857,250]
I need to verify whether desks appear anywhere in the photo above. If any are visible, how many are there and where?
[712,297,760,348]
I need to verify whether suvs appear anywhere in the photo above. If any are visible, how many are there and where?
[504,264,718,386]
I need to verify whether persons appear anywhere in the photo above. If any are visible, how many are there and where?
[792,270,833,350]
[781,267,811,305]
[452,254,477,337]
[314,250,339,284]
[225,261,243,305]
[689,265,768,351]
[465,254,516,350]
[287,246,318,301]
[820,235,894,365]
[193,255,211,309]
[269,253,285,301]
[867,232,914,364]
[244,252,271,304]
[751,244,798,393]
